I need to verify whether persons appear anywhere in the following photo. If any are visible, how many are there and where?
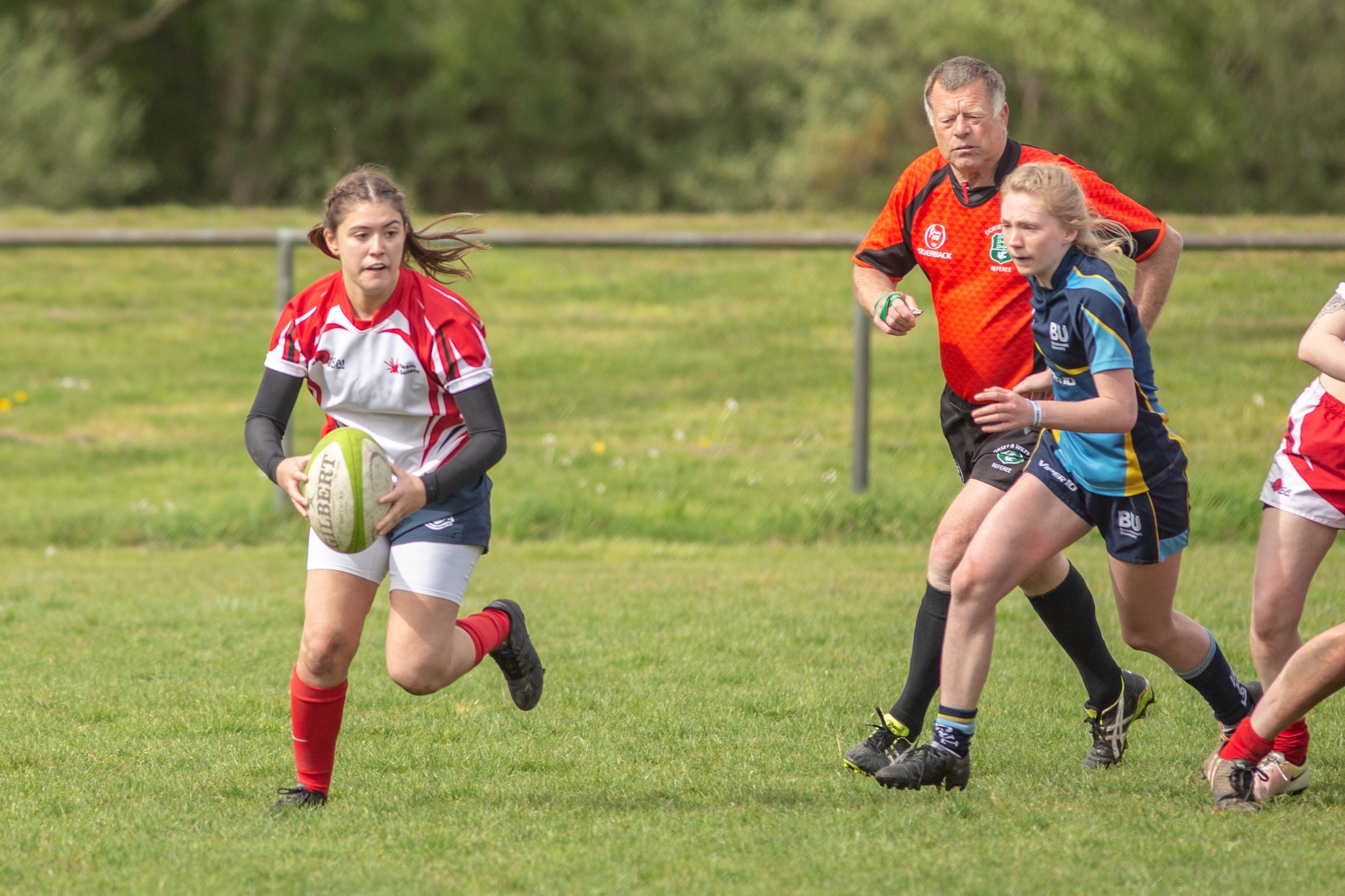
[849,54,1345,815]
[245,168,544,822]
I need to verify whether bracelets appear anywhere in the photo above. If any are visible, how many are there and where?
[871,293,890,309]
[1025,399,1042,430]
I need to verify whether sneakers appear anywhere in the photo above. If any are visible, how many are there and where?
[1252,748,1308,802]
[483,596,544,710]
[1202,749,1259,815]
[265,783,326,818]
[876,740,970,791]
[842,708,921,777]
[1217,682,1264,742]
[1084,670,1155,771]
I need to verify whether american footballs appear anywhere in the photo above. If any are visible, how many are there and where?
[302,426,393,555]
